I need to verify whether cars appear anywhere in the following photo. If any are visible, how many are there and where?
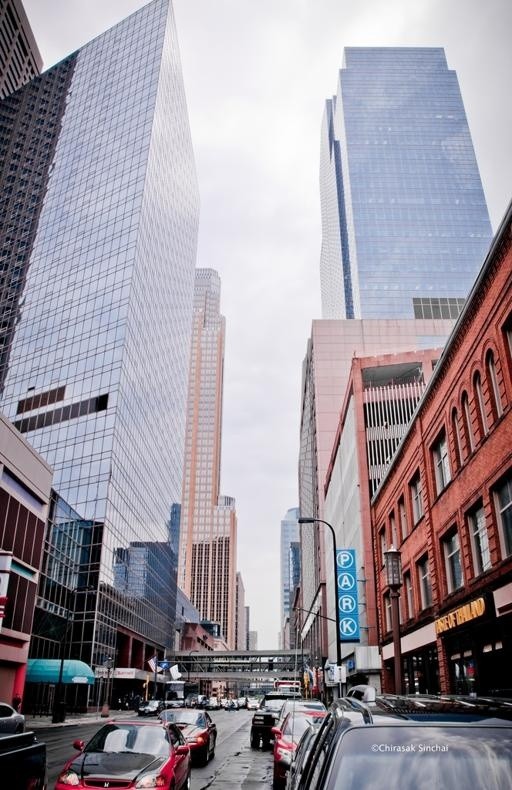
[1,681,512,790]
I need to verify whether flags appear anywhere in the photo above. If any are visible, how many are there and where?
[169,664,182,680]
[148,656,163,673]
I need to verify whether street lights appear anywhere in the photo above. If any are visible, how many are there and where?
[298,518,344,698]
[54,585,94,721]
[101,657,113,717]
[383,544,404,697]
